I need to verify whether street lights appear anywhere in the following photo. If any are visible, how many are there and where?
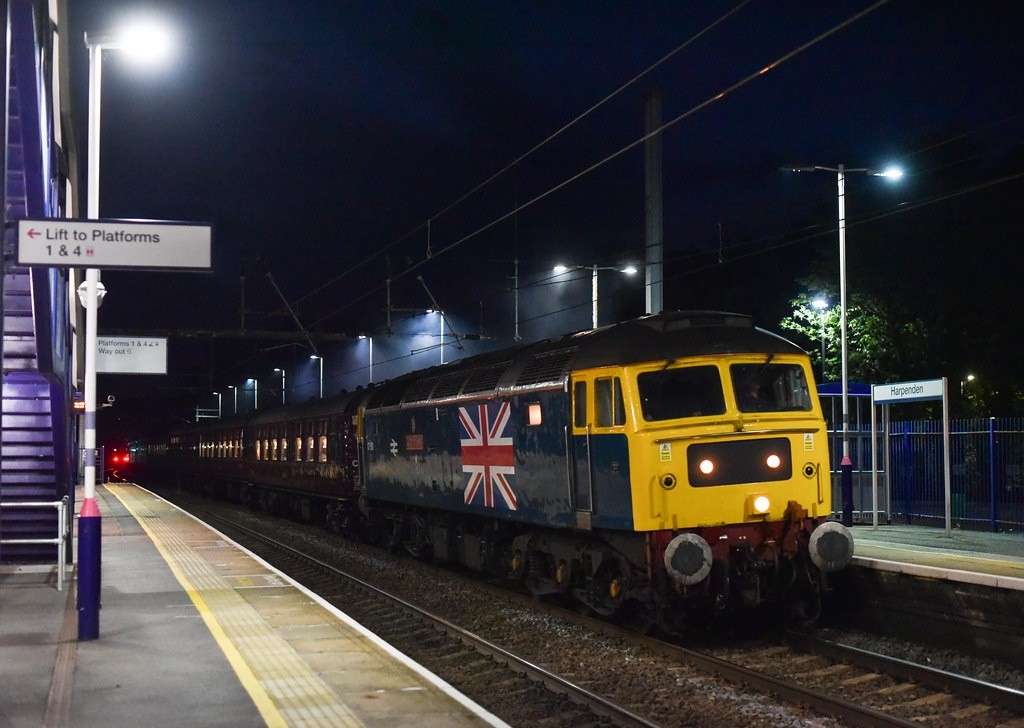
[226,386,238,416]
[358,335,373,385]
[779,161,906,521]
[274,368,286,405]
[960,375,974,400]
[247,377,258,411]
[310,354,323,399]
[213,391,222,420]
[76,24,174,629]
[548,264,637,327]
[425,310,447,365]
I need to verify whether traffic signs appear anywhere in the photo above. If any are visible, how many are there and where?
[12,216,214,274]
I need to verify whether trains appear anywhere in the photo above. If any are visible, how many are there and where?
[126,306,855,640]
